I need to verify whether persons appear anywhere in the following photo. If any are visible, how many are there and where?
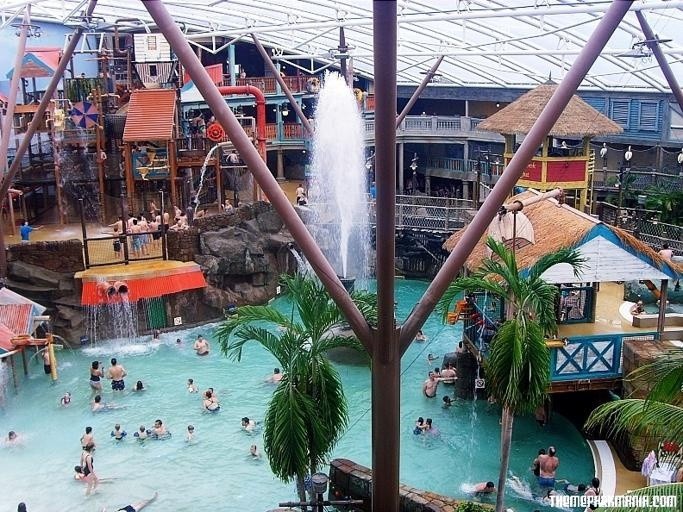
[392,190,683,512]
[0,56,379,512]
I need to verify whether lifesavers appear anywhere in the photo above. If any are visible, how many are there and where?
[207,124,224,141]
